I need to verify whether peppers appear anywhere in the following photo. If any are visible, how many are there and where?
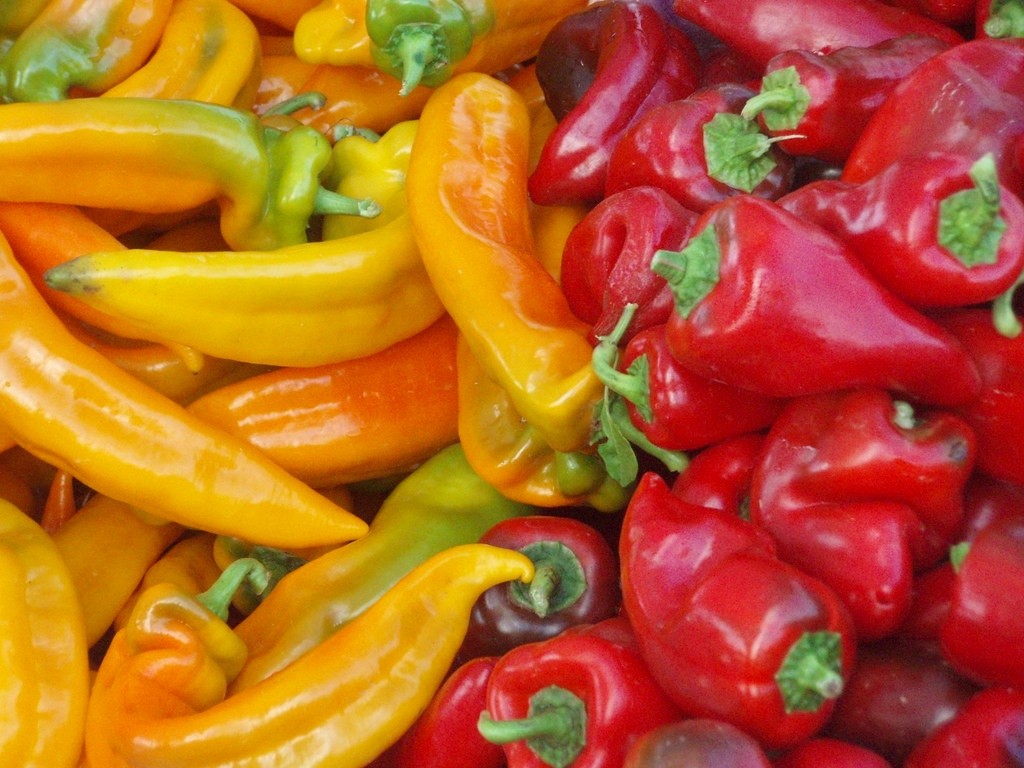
[0,0,1024,768]
